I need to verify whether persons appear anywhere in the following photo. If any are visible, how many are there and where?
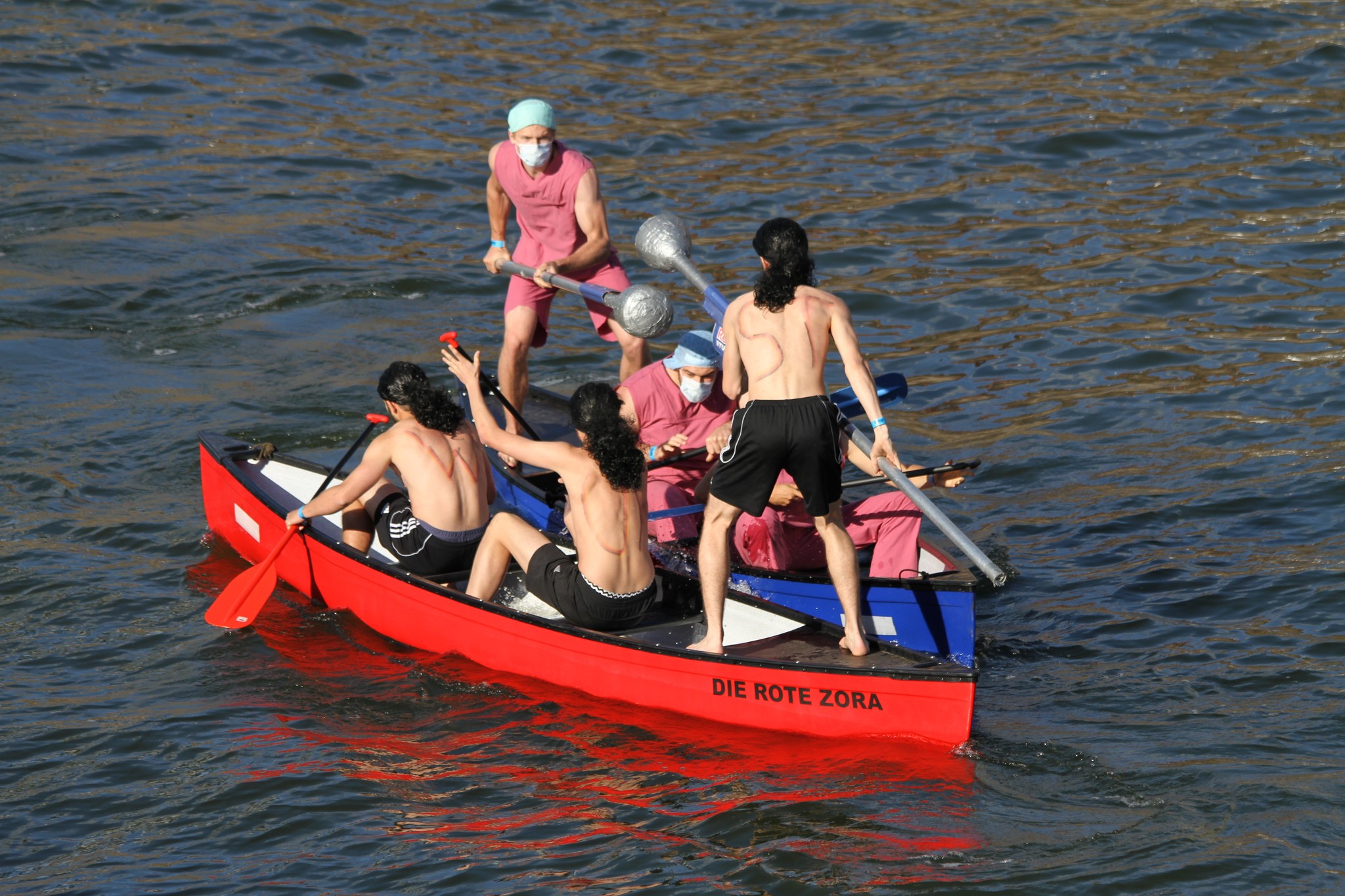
[482,98,651,467]
[440,344,658,633]
[688,217,902,657]
[611,329,975,579]
[286,362,495,578]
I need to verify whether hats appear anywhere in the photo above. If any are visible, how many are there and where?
[508,99,556,134]
[663,330,720,371]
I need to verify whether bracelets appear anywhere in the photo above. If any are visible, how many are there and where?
[870,417,886,429]
[490,240,507,248]
[649,446,658,461]
[929,474,937,487]
[298,505,309,520]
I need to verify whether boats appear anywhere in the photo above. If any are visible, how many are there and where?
[196,441,978,756]
[479,446,978,673]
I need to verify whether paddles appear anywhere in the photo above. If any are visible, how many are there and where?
[204,413,389,630]
[440,330,541,441]
[647,458,983,522]
[545,373,908,510]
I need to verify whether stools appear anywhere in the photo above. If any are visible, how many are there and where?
[384,556,524,583]
[548,601,704,635]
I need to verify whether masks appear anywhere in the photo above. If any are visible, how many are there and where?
[514,141,552,167]
[678,369,713,404]
